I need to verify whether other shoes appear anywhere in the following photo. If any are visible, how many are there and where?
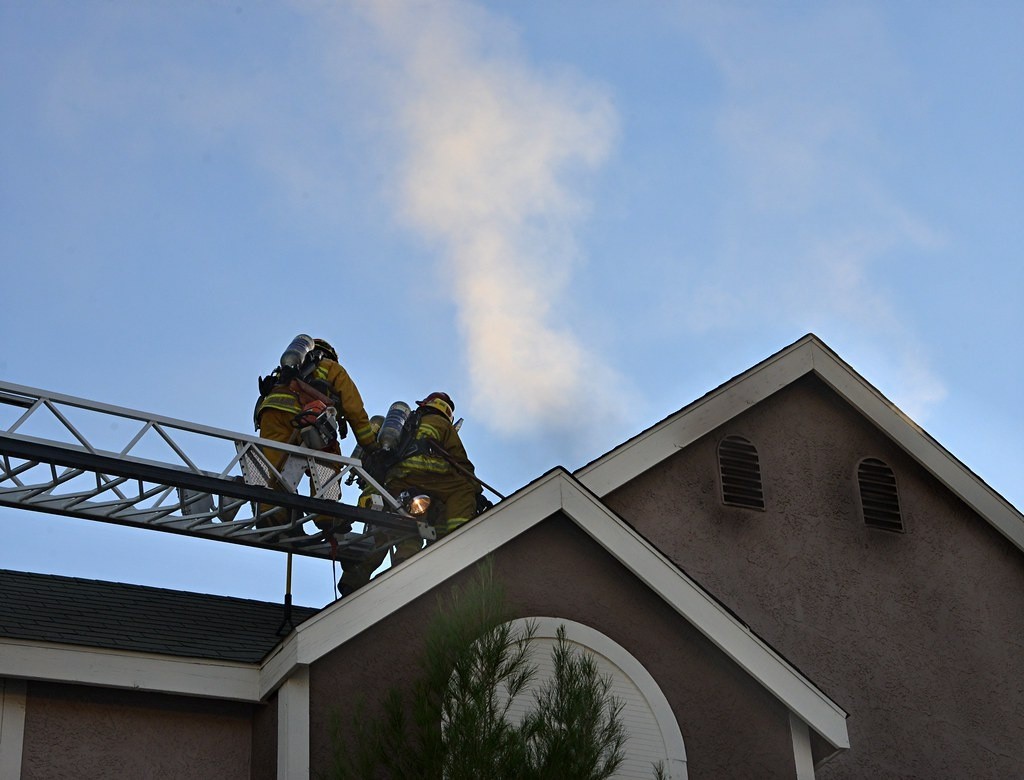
[256,519,279,543]
[317,520,352,534]
[338,583,353,595]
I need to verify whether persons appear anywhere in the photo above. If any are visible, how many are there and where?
[253,332,381,532]
[337,392,493,598]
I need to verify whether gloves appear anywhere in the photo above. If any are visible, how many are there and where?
[367,441,381,456]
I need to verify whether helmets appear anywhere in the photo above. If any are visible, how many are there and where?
[416,392,454,423]
[313,339,338,363]
